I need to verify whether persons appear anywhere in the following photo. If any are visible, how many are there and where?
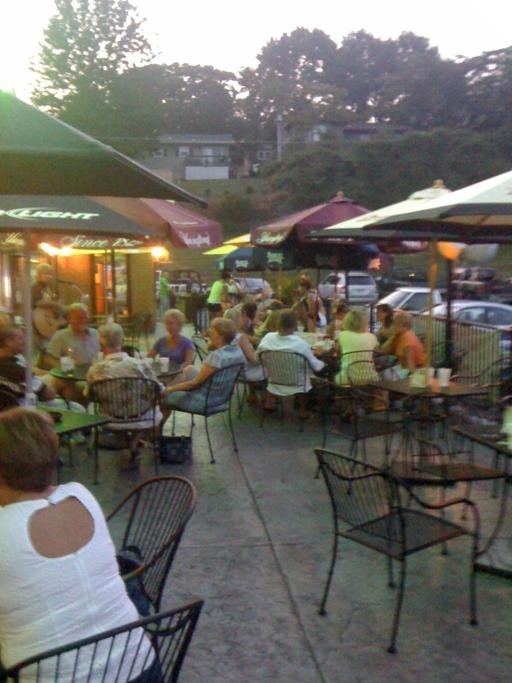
[146,309,195,405]
[159,318,245,425]
[87,323,165,471]
[43,302,103,408]
[30,263,64,363]
[206,271,425,428]
[0,407,163,683]
[0,324,86,442]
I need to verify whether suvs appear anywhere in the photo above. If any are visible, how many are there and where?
[318,271,376,303]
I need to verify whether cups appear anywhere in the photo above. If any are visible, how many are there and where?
[160,357,169,373]
[436,367,452,387]
[14,316,24,326]
[297,327,304,336]
[421,367,435,386]
[60,356,76,372]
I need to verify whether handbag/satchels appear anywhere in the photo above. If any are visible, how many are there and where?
[158,436,193,465]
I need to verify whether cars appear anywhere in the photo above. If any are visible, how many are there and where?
[230,277,276,300]
[369,284,512,351]
[154,270,206,304]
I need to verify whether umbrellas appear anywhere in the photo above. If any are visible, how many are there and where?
[362,169,512,246]
[307,179,457,372]
[203,233,395,303]
[250,191,429,331]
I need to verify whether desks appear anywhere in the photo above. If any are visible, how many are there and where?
[36,404,112,487]
[50,361,185,382]
[372,375,489,401]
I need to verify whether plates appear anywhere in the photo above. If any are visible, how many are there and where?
[48,411,62,420]
[312,340,335,350]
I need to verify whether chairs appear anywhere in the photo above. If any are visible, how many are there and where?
[91,376,163,484]
[397,356,511,577]
[339,349,389,391]
[51,309,153,361]
[256,349,332,434]
[0,599,205,682]
[313,448,481,653]
[433,349,473,384]
[348,360,404,479]
[167,361,248,466]
[236,373,285,429]
[303,373,392,497]
[189,333,266,418]
[186,292,256,336]
[106,472,198,612]
